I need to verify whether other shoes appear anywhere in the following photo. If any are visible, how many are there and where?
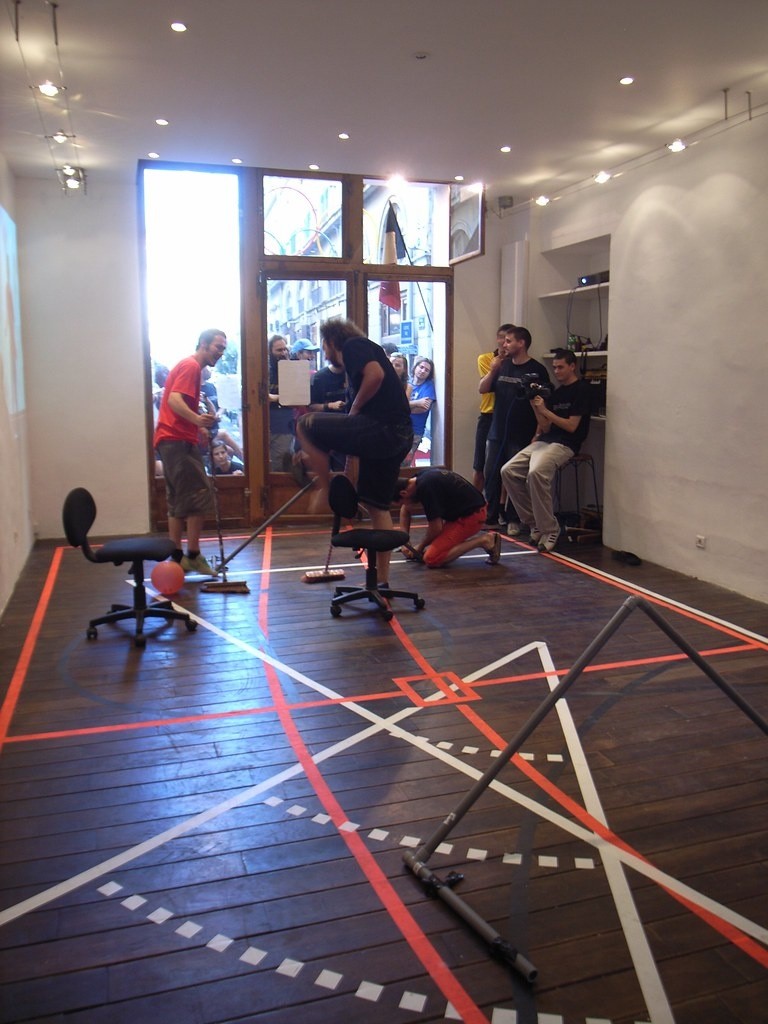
[507,522,521,536]
[368,582,390,602]
[497,512,508,525]
[308,487,330,515]
[480,523,500,529]
[168,556,192,574]
[180,552,218,576]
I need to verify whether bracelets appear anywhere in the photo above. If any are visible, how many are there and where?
[536,433,540,438]
[323,404,329,412]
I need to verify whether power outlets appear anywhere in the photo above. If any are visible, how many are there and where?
[695,535,706,549]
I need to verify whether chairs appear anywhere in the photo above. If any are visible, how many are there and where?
[327,474,430,622]
[61,486,197,640]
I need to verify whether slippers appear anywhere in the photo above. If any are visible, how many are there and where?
[483,532,502,565]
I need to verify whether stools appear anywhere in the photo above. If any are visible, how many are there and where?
[556,455,601,542]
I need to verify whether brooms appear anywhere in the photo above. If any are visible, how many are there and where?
[299,454,347,584]
[200,409,253,594]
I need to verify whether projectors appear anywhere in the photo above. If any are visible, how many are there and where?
[579,270,610,287]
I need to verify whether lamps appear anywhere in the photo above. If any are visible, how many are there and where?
[30,78,82,192]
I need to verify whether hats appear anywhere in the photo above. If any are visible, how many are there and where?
[290,338,320,355]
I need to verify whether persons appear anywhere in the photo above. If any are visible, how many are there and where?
[153,329,228,576]
[472,324,516,525]
[381,343,398,359]
[478,326,550,536]
[500,349,591,552]
[269,334,347,472]
[389,353,413,399]
[151,362,244,475]
[392,469,501,568]
[294,317,413,585]
[400,358,436,467]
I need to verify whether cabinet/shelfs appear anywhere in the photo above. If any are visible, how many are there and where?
[538,282,609,421]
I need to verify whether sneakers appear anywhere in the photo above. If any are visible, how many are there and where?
[537,526,561,553]
[527,525,541,545]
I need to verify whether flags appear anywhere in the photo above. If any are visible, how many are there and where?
[379,206,406,312]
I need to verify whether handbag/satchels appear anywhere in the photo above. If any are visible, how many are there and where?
[567,331,596,352]
